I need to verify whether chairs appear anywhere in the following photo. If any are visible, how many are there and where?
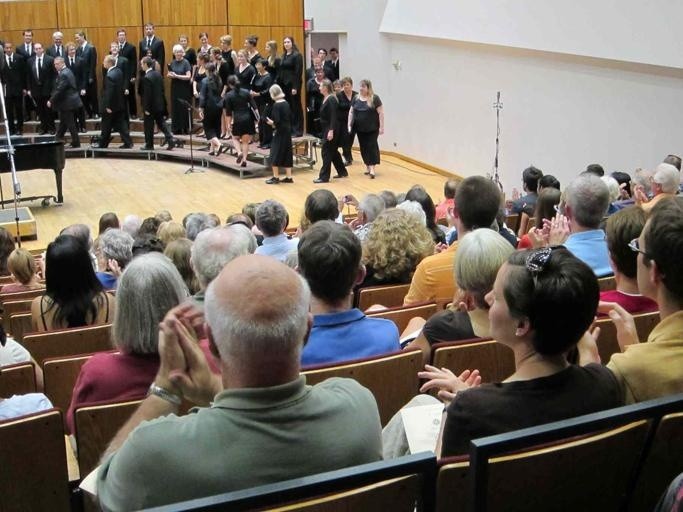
[0,204,683,512]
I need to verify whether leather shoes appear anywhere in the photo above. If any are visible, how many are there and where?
[38,128,87,149]
[313,161,352,182]
[92,138,178,150]
[196,132,253,166]
[266,177,293,184]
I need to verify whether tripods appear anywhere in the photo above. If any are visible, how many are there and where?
[184,108,205,174]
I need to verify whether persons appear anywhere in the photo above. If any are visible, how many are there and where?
[577,210,682,406]
[300,219,400,366]
[305,48,384,183]
[1,155,682,333]
[2,22,304,184]
[67,251,222,434]
[399,228,516,350]
[97,254,383,512]
[383,244,622,458]
[1,307,44,393]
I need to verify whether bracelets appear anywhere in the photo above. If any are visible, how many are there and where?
[145,383,182,404]
[443,407,446,412]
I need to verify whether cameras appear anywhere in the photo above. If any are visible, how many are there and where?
[343,196,350,202]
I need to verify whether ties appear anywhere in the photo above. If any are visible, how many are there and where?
[9,45,74,74]
[148,39,151,47]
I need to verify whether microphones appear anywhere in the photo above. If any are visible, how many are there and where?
[496,92,500,102]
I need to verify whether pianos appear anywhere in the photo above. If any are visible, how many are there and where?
[0,136,65,207]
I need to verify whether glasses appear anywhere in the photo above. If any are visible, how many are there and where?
[628,238,648,257]
[525,245,567,288]
